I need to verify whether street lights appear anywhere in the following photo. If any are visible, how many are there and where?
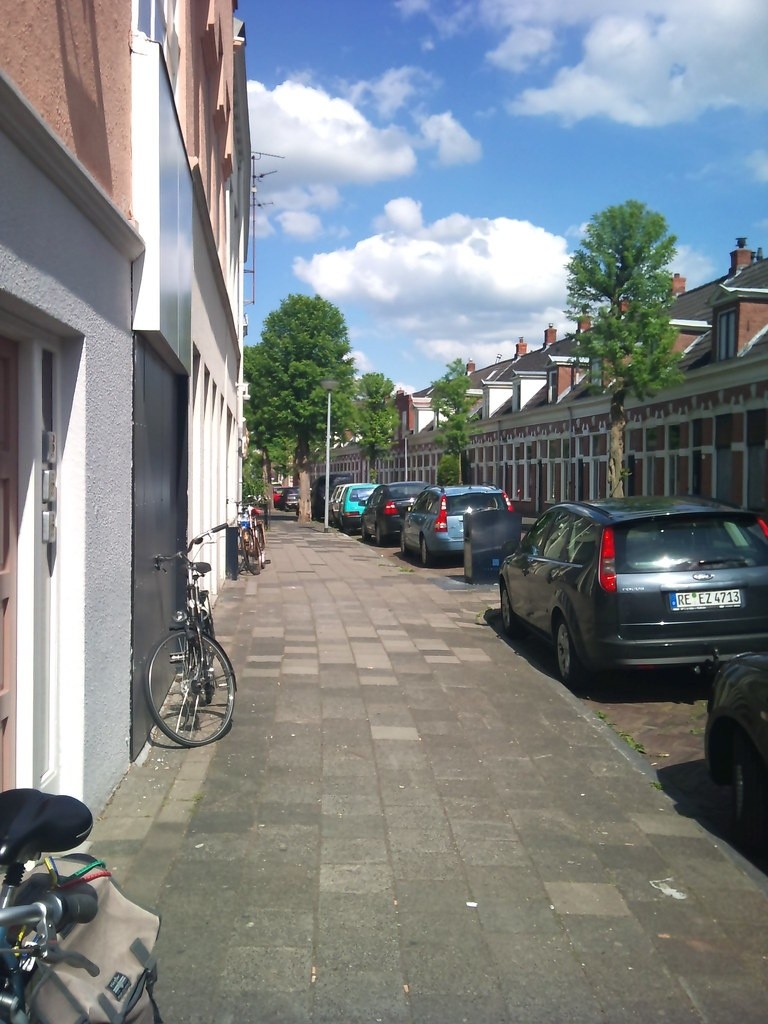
[318,380,341,533]
[400,429,411,482]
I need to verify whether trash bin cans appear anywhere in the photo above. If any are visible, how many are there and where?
[463,507,523,584]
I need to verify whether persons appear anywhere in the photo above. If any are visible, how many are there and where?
[273,490,281,508]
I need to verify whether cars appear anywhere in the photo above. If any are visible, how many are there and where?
[358,481,435,547]
[699,648,767,862]
[498,497,768,689]
[271,471,385,533]
[399,484,514,568]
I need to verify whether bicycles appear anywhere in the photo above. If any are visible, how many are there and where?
[236,494,271,576]
[141,523,239,749]
[0,788,110,1024]
